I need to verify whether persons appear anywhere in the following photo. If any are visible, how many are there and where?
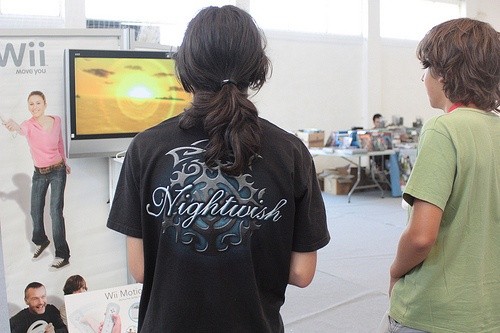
[9,282,68,333]
[370,114,382,129]
[6,91,71,270]
[384,18,500,333]
[125,5,316,333]
[59,275,88,329]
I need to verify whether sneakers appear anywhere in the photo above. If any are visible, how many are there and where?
[34,240,50,257]
[50,257,70,271]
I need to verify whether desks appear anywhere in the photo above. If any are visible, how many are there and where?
[310,148,396,203]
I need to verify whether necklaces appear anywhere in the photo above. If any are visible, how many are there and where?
[447,100,477,113]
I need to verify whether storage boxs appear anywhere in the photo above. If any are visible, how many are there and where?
[298,131,325,148]
[324,164,351,175]
[325,174,352,196]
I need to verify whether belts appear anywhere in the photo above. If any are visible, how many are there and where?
[36,161,64,175]
